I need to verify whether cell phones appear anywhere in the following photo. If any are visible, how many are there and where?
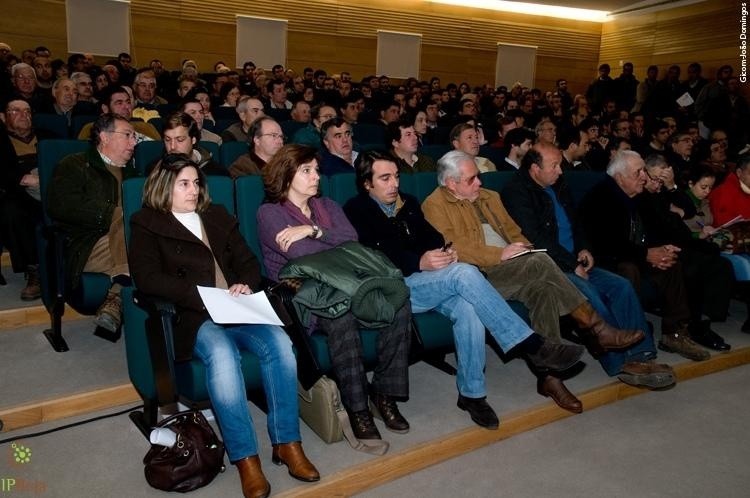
[442,241,453,252]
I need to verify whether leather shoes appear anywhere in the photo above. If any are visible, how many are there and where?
[527,342,585,412]
[595,324,731,390]
[272,442,320,481]
[457,393,498,429]
[236,454,270,498]
[350,394,409,439]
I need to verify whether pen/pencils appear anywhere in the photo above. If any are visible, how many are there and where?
[696,220,705,228]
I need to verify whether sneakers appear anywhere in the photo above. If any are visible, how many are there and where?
[94,289,123,332]
[21,264,41,299]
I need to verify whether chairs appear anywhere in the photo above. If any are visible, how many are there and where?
[0,68,750,445]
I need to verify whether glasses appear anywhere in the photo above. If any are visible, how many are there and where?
[461,176,475,185]
[646,171,664,183]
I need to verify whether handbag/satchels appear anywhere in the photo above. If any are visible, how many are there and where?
[299,375,343,443]
[143,409,225,493]
